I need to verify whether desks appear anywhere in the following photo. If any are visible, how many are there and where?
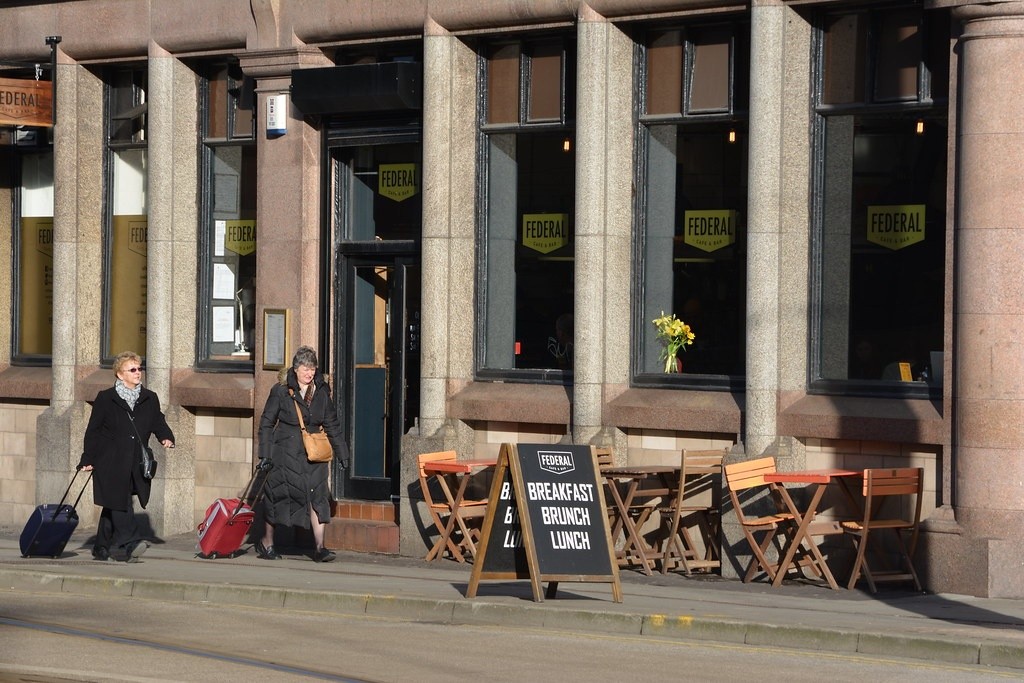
[766,470,863,589]
[424,461,498,564]
[600,466,681,575]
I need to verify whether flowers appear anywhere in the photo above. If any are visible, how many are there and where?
[652,310,695,365]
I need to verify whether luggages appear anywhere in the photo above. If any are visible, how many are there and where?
[19,464,95,559]
[196,468,270,560]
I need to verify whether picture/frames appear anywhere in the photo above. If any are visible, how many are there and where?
[262,309,291,372]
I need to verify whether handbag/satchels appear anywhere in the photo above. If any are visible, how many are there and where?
[301,426,333,462]
[141,445,158,480]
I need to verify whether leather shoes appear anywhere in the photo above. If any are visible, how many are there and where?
[313,545,337,562]
[256,538,282,561]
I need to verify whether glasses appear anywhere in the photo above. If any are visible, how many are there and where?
[122,367,143,373]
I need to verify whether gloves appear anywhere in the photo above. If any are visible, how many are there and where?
[259,457,274,471]
[339,459,349,472]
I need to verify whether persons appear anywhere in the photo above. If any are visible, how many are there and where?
[77,351,175,563]
[257,346,350,561]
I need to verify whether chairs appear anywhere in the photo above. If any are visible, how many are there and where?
[416,450,925,597]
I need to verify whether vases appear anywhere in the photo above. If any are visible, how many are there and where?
[665,345,678,374]
